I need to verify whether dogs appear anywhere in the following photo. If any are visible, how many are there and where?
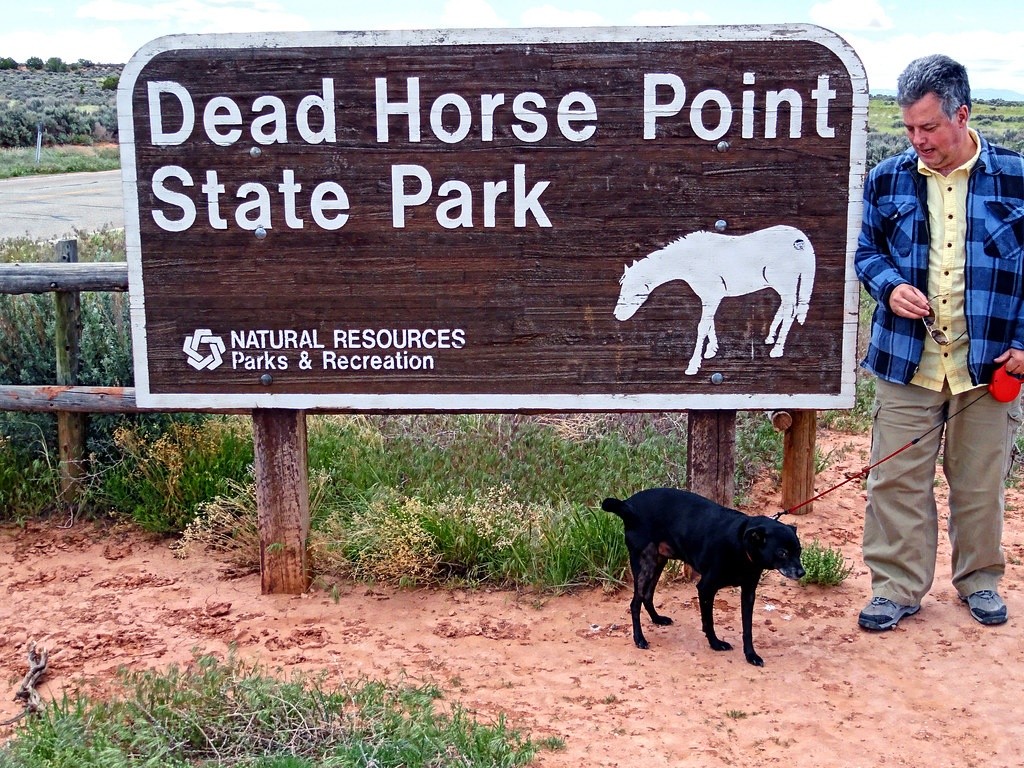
[601,488,806,667]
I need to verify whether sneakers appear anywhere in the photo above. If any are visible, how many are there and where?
[858,596,921,630]
[957,588,1008,625]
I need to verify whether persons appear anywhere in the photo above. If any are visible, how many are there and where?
[851,53,1024,634]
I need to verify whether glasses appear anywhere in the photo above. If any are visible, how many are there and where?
[921,293,967,346]
